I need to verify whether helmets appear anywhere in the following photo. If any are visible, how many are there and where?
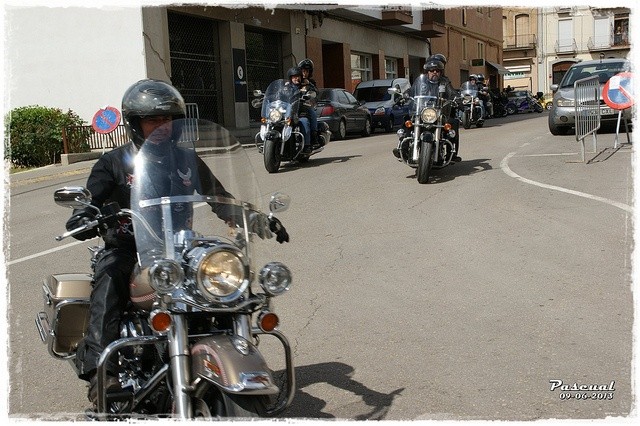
[122,78,187,157]
[297,58,314,78]
[287,66,304,83]
[469,73,478,81]
[425,53,447,65]
[477,73,484,82]
[423,60,445,77]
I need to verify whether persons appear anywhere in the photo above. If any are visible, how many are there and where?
[426,54,455,91]
[468,73,487,122]
[65,78,289,421]
[252,68,317,153]
[297,58,320,152]
[477,73,491,119]
[392,61,480,165]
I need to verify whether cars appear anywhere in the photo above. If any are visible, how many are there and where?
[549,59,631,135]
[506,90,534,104]
[314,88,373,141]
[537,92,555,110]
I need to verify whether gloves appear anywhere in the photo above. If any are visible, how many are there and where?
[66,208,102,241]
[250,214,289,244]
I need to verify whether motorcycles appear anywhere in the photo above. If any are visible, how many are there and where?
[387,75,464,184]
[252,79,332,173]
[490,92,508,118]
[504,90,543,115]
[35,118,295,421]
[455,81,486,129]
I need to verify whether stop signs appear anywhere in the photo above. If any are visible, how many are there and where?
[602,72,634,109]
[92,105,121,134]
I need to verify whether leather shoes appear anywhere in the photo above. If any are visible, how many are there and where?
[85,375,122,405]
[303,145,313,153]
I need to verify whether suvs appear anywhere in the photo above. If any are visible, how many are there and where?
[352,78,412,133]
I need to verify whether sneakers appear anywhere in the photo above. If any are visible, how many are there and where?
[310,134,320,147]
[445,153,461,161]
[393,148,409,160]
